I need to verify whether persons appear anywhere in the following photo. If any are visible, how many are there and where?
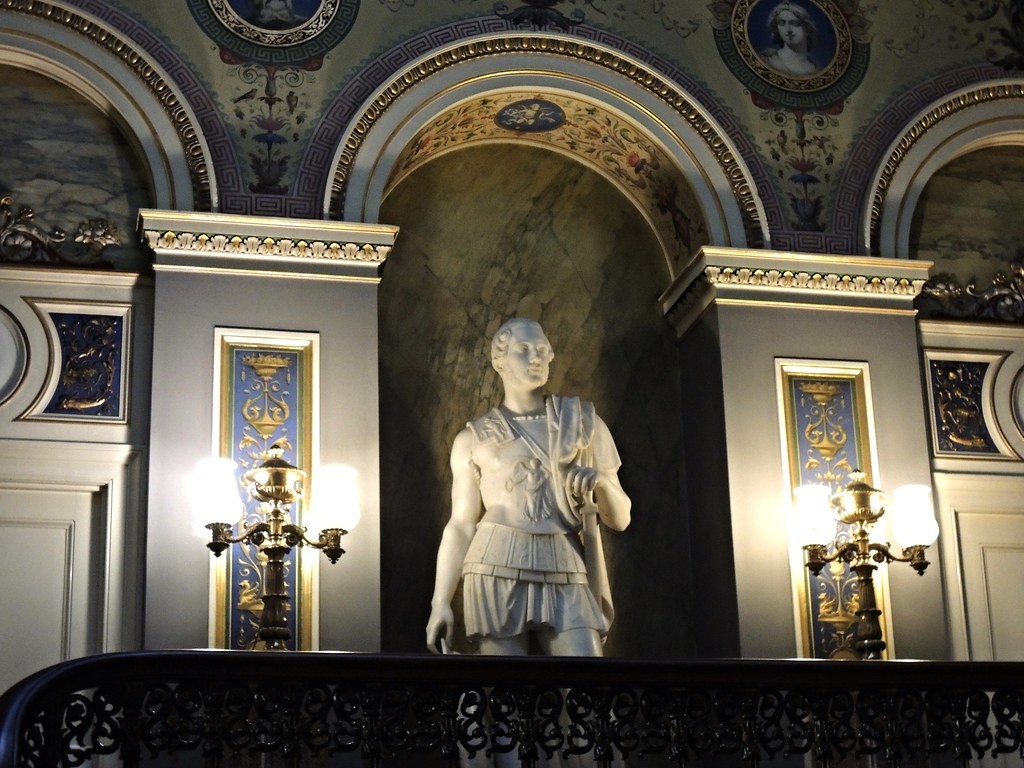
[426,318,636,768]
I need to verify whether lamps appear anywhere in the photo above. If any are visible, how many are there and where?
[195,444,360,657]
[792,468,941,660]
